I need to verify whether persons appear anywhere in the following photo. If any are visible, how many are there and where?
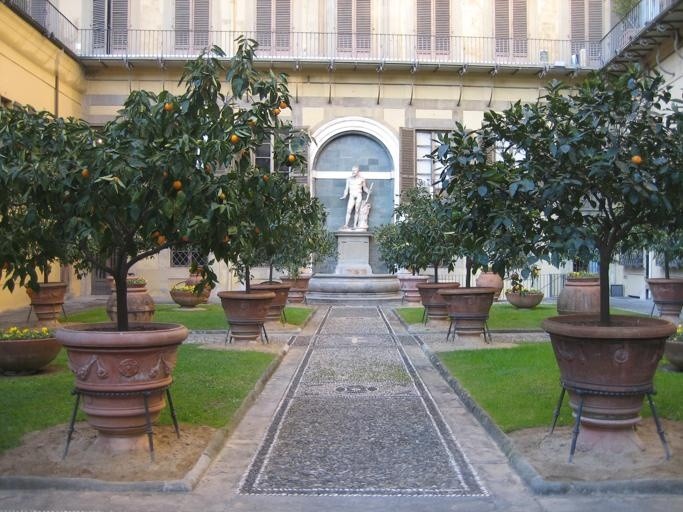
[340,165,372,228]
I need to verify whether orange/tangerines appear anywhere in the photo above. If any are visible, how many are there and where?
[632,155,641,164]
[79,101,297,247]
